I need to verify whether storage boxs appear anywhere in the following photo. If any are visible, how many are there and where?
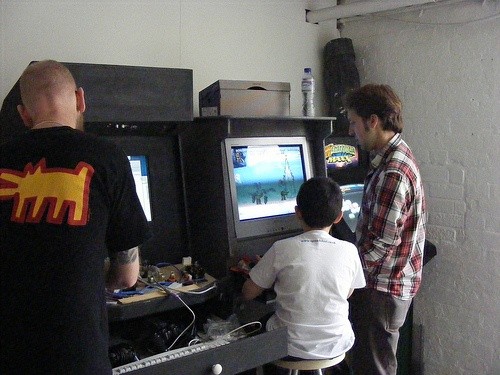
[199,80,290,118]
[3,61,193,122]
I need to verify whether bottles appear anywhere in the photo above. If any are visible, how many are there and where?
[300,67,315,117]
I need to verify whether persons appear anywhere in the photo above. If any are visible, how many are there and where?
[243,176,366,375]
[346,83,426,375]
[0,60,153,375]
[235,152,244,163]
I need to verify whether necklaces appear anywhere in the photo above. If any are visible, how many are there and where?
[31,119,65,128]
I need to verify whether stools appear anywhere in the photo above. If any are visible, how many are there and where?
[273,353,346,375]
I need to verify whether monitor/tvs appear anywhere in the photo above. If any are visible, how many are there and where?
[332,178,366,244]
[99,135,183,265]
[222,136,314,240]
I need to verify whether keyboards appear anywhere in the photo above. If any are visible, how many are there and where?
[111,337,239,375]
